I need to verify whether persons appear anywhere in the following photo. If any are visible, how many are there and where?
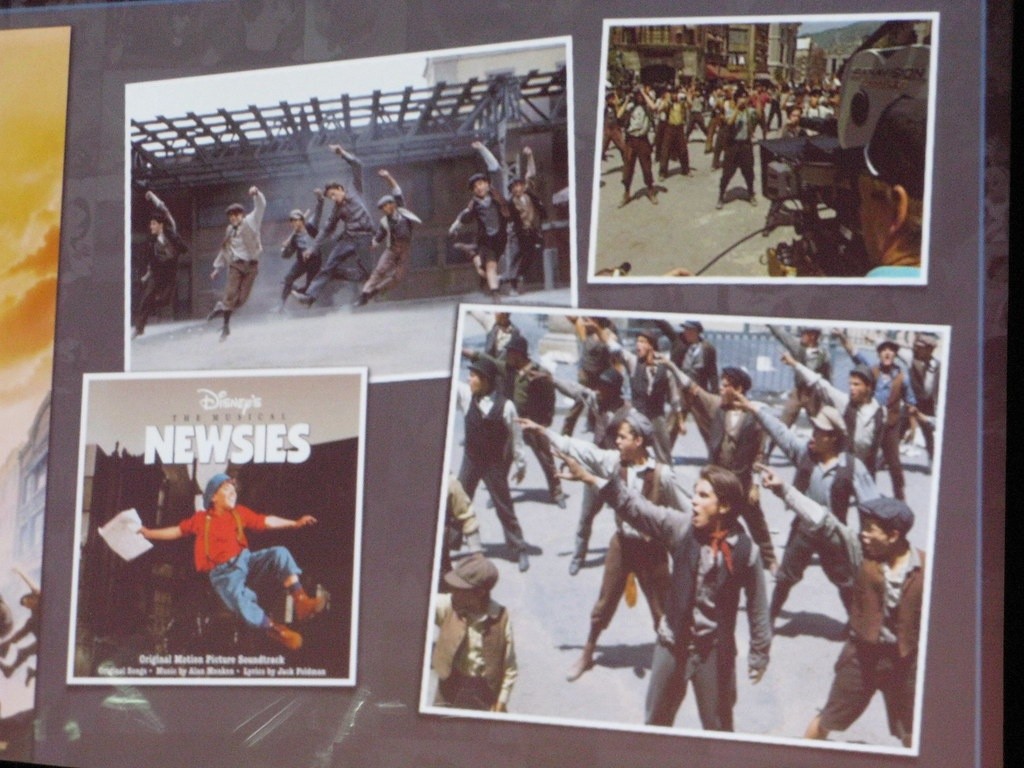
[137,74,941,749]
[0,565,43,691]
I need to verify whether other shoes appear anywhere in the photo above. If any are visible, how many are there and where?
[568,660,592,681]
[570,558,584,574]
[294,597,325,618]
[559,499,565,509]
[278,625,303,649]
[518,553,527,572]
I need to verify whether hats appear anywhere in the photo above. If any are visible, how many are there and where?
[877,340,900,354]
[624,412,656,445]
[505,336,530,356]
[289,210,303,221]
[378,194,393,209]
[724,367,752,388]
[854,495,913,536]
[468,173,487,190]
[850,363,875,391]
[203,474,230,509]
[594,367,624,395]
[508,178,523,191]
[681,320,705,334]
[832,97,927,201]
[810,405,847,437]
[225,204,244,214]
[914,335,936,350]
[444,554,498,592]
[467,360,498,386]
[636,330,659,347]
